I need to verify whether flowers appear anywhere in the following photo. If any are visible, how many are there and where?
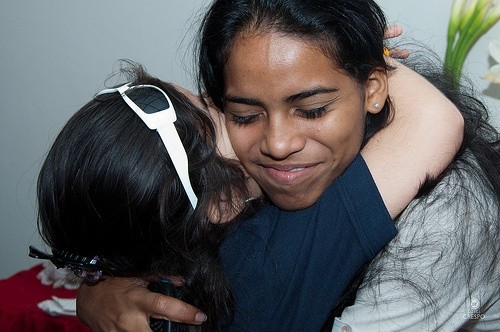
[443,0,500,88]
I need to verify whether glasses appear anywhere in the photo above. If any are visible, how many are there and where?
[94,80,198,212]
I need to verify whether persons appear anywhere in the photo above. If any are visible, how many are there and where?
[27,23,468,332]
[73,0,500,332]
